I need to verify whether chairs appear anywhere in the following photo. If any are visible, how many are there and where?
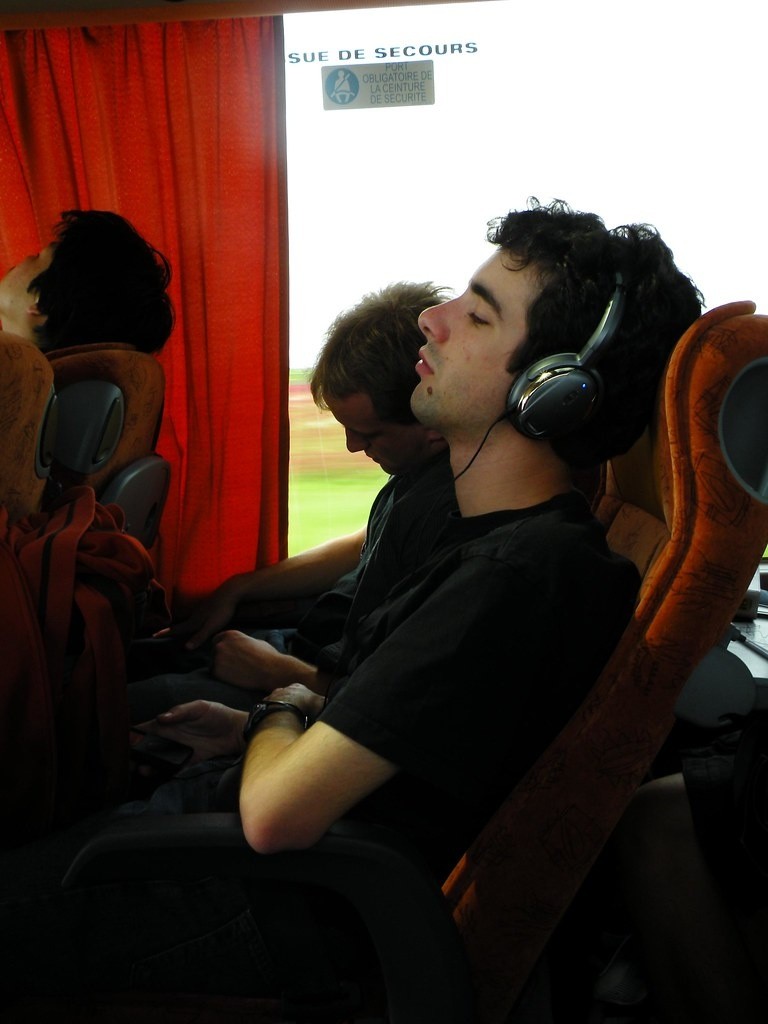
[10,340,171,769]
[59,304,768,1024]
[0,329,61,844]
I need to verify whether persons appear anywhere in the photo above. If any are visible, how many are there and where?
[126,286,444,727]
[0,209,175,355]
[1,205,701,996]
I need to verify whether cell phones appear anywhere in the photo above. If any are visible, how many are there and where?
[129,727,193,770]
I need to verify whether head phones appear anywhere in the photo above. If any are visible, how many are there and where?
[505,270,633,443]
